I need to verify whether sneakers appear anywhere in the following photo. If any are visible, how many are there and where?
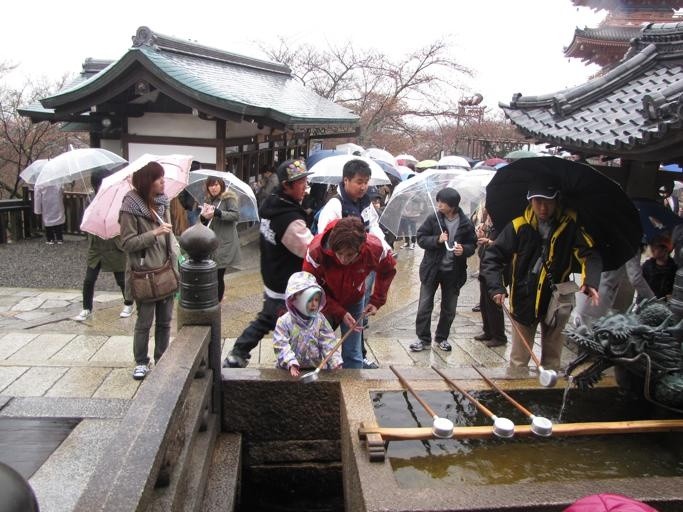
[75,310,93,320]
[363,359,378,368]
[410,340,430,352]
[472,303,480,311]
[399,242,415,250]
[472,270,480,278]
[475,334,505,347]
[437,341,451,351]
[224,354,249,368]
[133,364,148,378]
[120,304,134,317]
[45,240,63,245]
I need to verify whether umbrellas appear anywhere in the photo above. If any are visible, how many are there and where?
[19,158,71,185]
[34,143,128,203]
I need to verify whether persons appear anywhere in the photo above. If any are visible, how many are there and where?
[222,156,318,368]
[32,179,65,245]
[477,175,604,380]
[250,153,510,252]
[198,173,243,308]
[297,215,399,370]
[76,164,137,326]
[563,144,681,382]
[408,185,478,353]
[118,157,183,381]
[268,271,343,377]
[307,157,386,370]
[470,190,512,349]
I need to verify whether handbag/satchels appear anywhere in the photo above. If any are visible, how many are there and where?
[545,282,579,329]
[129,260,178,304]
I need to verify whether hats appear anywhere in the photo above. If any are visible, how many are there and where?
[291,287,321,317]
[527,180,561,199]
[277,158,314,181]
[650,235,673,250]
